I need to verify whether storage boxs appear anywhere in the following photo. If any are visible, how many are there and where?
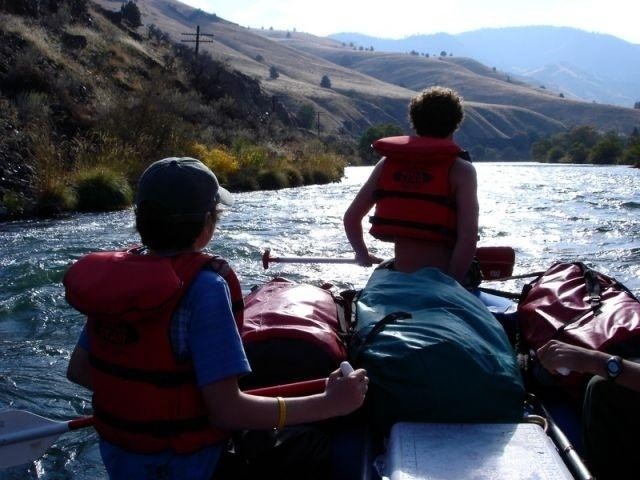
[378,418,574,480]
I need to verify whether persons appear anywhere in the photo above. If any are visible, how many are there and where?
[62,156,370,479]
[536,339,640,467]
[343,86,478,282]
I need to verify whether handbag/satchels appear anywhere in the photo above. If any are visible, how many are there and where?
[518,261,639,424]
[346,262,525,424]
[241,278,346,396]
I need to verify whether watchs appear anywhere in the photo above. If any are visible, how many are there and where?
[605,355,623,382]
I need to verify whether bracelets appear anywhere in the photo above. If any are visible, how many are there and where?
[272,397,286,435]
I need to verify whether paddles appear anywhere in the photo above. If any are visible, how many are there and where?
[262,246,515,280]
[0,360,354,468]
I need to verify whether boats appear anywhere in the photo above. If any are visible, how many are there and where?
[246,283,640,479]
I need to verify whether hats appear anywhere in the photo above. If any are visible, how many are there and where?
[135,156,234,206]
